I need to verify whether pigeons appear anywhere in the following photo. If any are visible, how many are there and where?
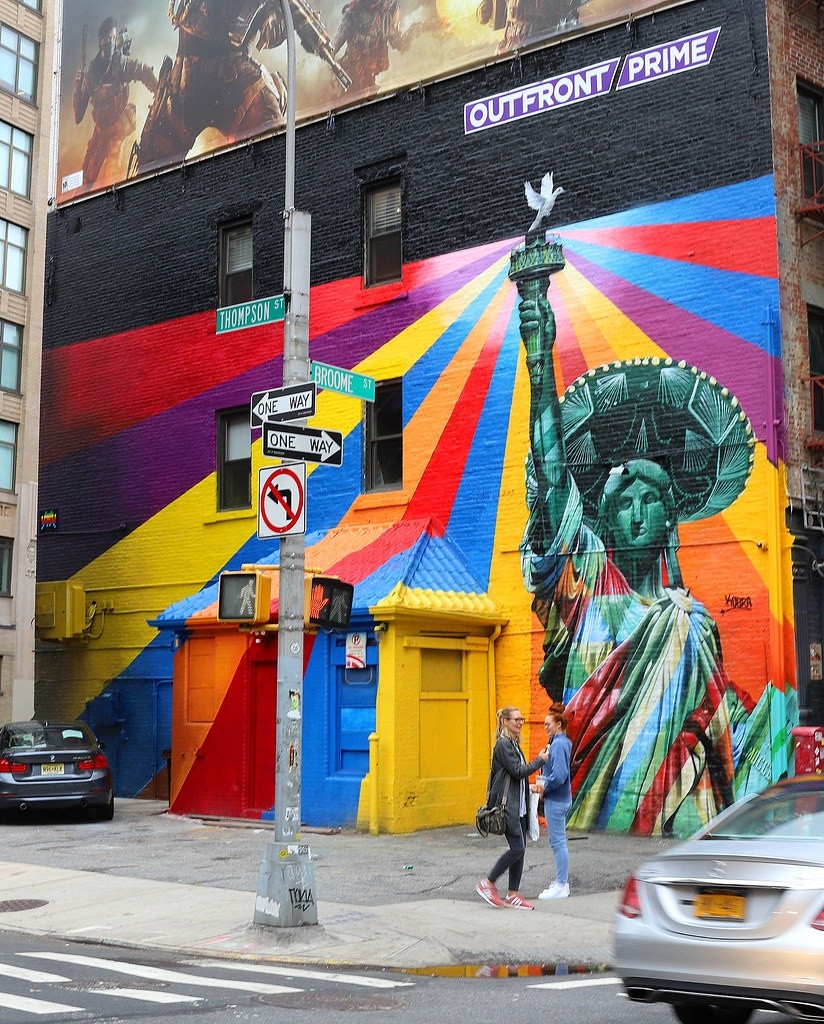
[525,170,565,233]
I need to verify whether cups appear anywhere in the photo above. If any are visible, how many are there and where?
[535,775,546,792]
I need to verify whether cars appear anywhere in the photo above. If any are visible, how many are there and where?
[610,771,823,1024]
[0,718,114,821]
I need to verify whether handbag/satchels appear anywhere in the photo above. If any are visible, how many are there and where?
[476,804,506,837]
[528,784,540,842]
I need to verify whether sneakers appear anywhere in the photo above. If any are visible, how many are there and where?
[475,881,504,907]
[538,882,570,900]
[505,895,534,910]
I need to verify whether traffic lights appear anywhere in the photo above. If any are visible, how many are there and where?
[216,570,272,626]
[303,572,355,631]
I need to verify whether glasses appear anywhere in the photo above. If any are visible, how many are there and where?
[505,718,525,723]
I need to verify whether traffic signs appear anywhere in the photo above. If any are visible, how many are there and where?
[250,379,315,430]
[260,418,344,468]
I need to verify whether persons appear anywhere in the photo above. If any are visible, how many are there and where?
[476,707,549,912]
[532,704,574,900]
[72,0,624,189]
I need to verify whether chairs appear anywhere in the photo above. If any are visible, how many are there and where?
[63,737,83,744]
[11,740,32,746]
